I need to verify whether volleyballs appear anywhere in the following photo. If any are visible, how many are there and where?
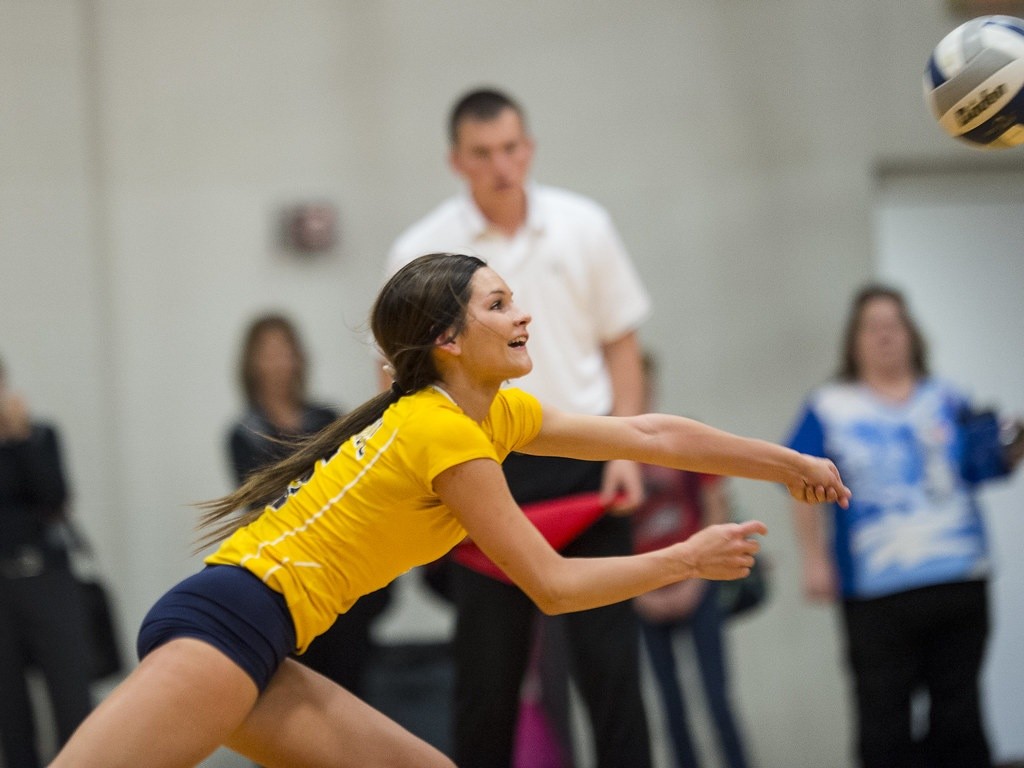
[918,13,1024,152]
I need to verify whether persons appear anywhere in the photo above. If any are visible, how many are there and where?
[48,251,852,768]
[227,311,373,703]
[0,362,96,768]
[785,282,1023,768]
[377,81,760,768]
